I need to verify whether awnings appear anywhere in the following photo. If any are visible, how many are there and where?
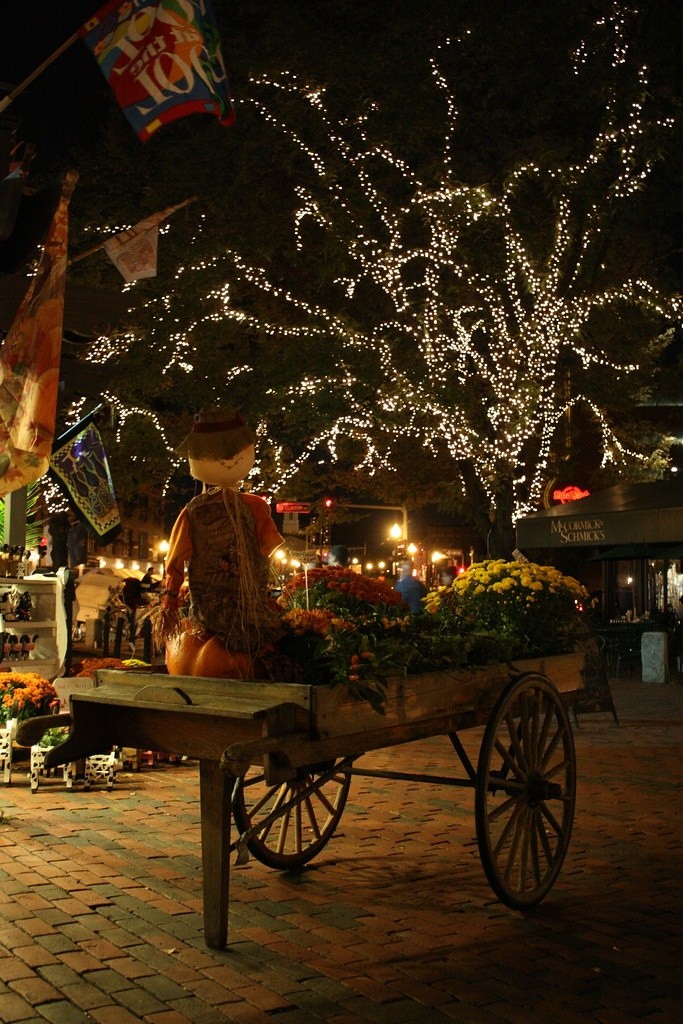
[514,482,682,546]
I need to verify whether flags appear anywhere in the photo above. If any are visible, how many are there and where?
[1,191,71,505]
[79,0,235,141]
[101,200,190,283]
[43,414,122,546]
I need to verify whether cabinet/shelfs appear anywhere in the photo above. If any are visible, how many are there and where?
[0,576,61,667]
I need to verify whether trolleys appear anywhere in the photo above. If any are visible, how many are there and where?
[13,649,587,948]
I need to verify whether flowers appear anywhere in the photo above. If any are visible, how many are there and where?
[276,559,596,700]
[69,657,152,678]
[0,671,65,718]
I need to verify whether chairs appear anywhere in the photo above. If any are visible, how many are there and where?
[597,612,683,680]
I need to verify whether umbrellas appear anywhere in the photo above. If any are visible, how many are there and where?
[585,544,683,619]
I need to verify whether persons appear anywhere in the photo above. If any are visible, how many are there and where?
[441,565,457,586]
[149,404,287,682]
[327,545,350,570]
[396,562,428,614]
